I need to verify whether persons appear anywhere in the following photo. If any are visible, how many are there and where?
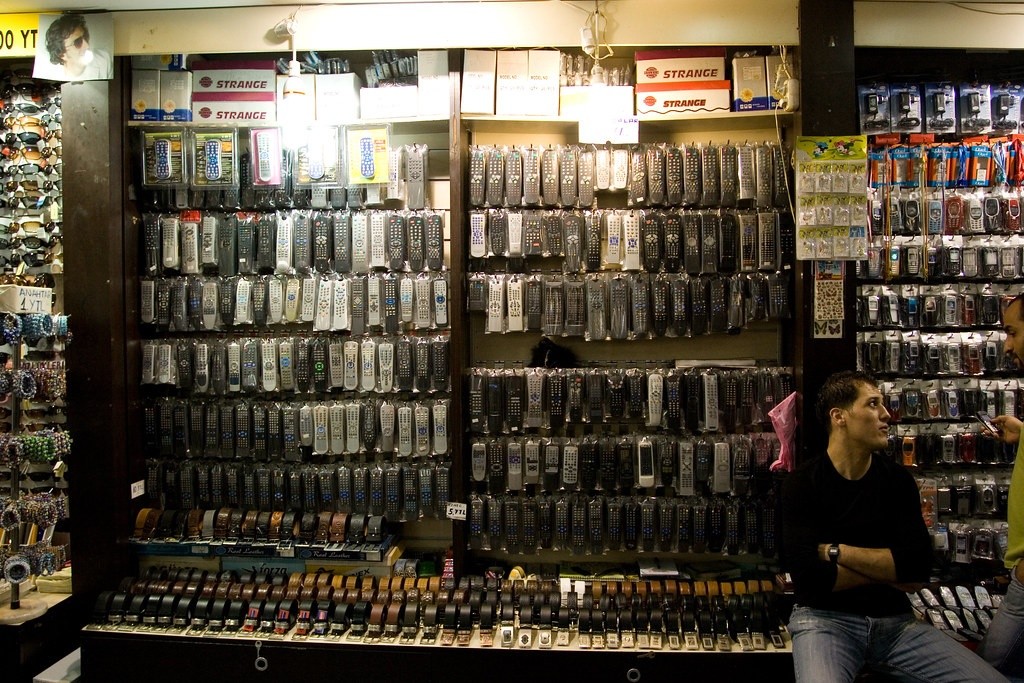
[975,292,1023,683]
[46,14,113,81]
[782,370,1009,683]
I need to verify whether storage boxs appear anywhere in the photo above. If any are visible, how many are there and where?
[123,48,809,125]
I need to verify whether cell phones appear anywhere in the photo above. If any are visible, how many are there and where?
[975,410,1004,436]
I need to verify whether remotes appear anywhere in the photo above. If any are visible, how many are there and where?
[139,135,798,558]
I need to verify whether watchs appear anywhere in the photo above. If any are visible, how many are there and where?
[827,543,839,561]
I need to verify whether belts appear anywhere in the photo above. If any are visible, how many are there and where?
[130,508,385,554]
[95,562,787,651]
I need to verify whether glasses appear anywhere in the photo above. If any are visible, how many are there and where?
[64,35,90,49]
[0,68,62,288]
